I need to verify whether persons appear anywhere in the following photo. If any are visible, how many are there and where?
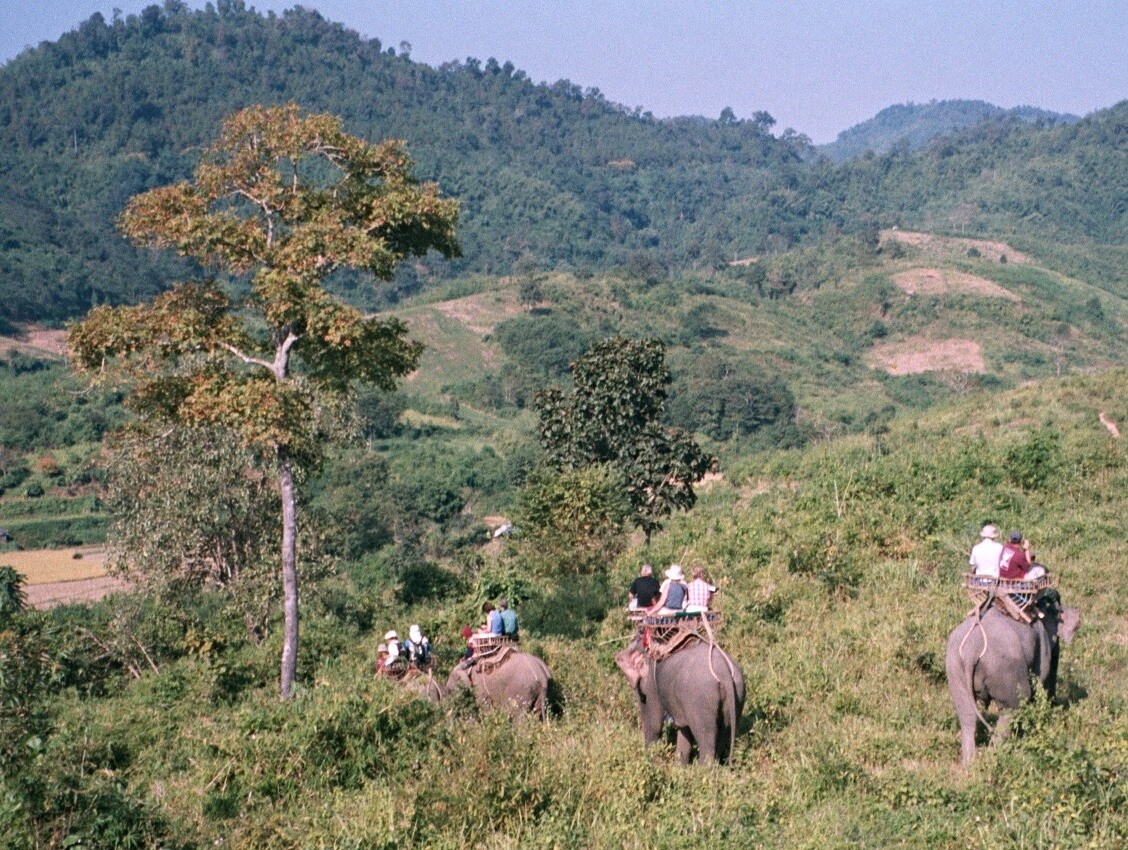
[375,624,431,676]
[998,530,1049,581]
[627,563,719,616]
[968,525,1004,584]
[460,598,520,662]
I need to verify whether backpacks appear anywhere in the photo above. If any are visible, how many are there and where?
[408,636,426,664]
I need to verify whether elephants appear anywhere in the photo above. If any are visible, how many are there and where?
[374,651,553,723]
[945,587,1081,770]
[615,626,749,768]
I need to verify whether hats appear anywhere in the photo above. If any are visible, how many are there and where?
[664,564,684,580]
[1010,531,1022,543]
[979,525,999,539]
[384,630,398,640]
[377,644,388,652]
